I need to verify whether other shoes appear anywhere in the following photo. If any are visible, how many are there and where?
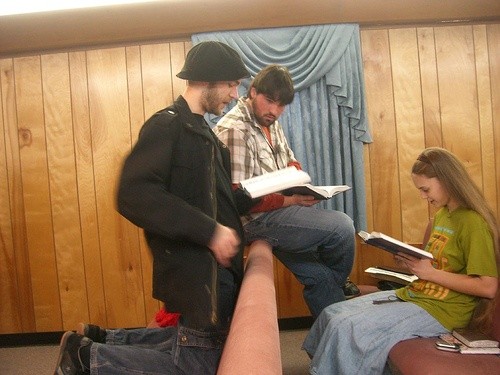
[343,279,361,299]
[53,331,93,375]
[76,322,106,342]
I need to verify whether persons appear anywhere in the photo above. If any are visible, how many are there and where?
[53,41,264,375]
[300,146,500,375]
[211,63,361,320]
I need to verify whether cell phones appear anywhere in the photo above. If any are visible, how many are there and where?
[435,341,462,352]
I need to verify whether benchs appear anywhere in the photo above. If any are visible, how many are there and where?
[215,240,283,375]
[358,217,500,375]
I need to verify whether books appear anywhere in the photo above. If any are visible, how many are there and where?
[439,328,500,354]
[238,166,311,199]
[357,230,433,260]
[280,183,352,199]
[364,265,418,286]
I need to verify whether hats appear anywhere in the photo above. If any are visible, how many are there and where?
[176,41,253,82]
